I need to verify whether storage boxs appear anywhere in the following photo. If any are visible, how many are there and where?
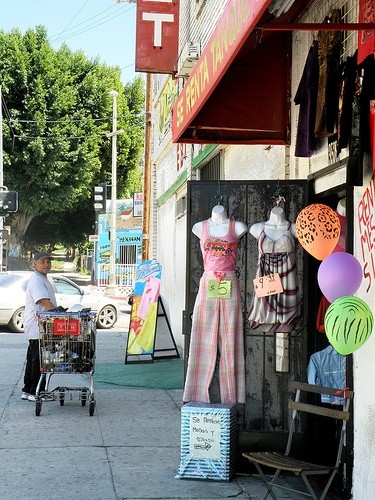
[179,401,236,483]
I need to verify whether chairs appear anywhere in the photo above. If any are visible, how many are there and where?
[242,381,354,500]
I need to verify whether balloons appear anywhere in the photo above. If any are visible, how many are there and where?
[296,204,374,356]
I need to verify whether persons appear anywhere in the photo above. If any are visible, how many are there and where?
[21,252,56,401]
[192,193,248,242]
[249,200,298,240]
[337,198,346,217]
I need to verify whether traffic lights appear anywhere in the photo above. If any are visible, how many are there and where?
[94,182,106,214]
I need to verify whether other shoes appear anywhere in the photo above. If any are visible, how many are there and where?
[21,390,55,401]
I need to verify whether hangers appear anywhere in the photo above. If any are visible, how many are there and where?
[326,6,333,17]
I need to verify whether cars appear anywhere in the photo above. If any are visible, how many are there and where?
[0,270,119,334]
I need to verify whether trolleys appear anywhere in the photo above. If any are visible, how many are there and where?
[34,306,97,417]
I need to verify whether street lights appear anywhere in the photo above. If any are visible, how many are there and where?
[105,89,119,296]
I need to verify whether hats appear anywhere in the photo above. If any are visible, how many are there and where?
[34,252,54,260]
